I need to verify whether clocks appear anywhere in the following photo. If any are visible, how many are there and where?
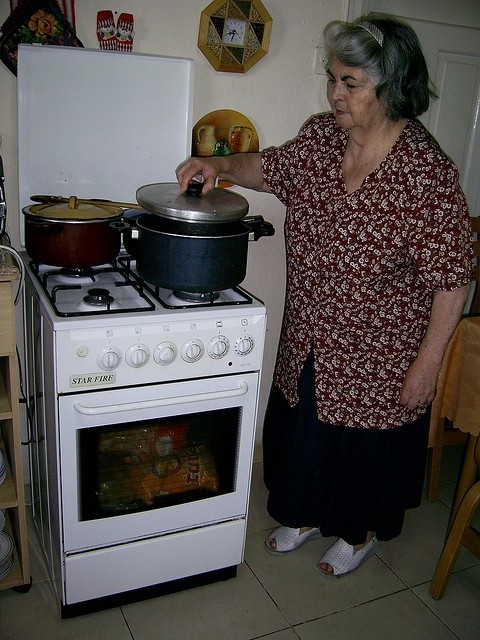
[197,0,273,73]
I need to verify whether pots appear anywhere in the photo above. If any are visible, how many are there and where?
[124,208,147,257]
[136,183,275,292]
[21,204,124,268]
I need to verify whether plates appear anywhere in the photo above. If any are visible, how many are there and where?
[192,109,259,188]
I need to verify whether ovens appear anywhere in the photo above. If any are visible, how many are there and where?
[40,316,262,619]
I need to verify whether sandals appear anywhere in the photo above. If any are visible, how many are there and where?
[315,532,379,579]
[265,525,321,556]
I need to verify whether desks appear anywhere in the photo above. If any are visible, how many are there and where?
[429,315,480,547]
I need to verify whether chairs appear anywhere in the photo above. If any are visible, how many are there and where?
[460,214,479,316]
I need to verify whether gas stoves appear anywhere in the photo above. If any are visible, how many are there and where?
[21,249,266,322]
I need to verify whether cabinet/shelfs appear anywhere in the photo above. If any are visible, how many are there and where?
[0,265,31,595]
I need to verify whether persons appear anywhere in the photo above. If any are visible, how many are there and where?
[174,12,478,580]
